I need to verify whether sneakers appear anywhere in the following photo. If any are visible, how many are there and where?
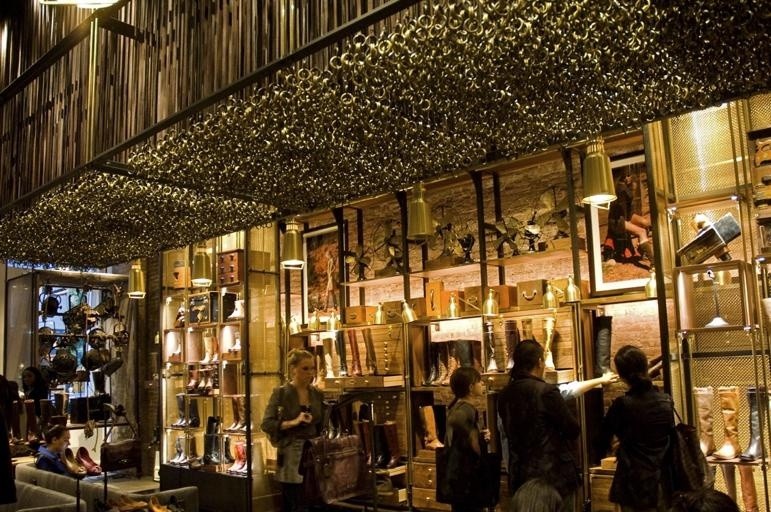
[62,447,87,477]
[93,494,184,512]
[76,447,102,475]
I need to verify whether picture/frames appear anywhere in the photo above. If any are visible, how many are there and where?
[299,221,348,331]
[583,149,659,298]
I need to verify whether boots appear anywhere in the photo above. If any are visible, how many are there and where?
[506,317,557,370]
[203,434,235,467]
[171,393,200,427]
[592,316,615,376]
[226,442,263,476]
[12,393,69,444]
[227,300,244,318]
[172,344,180,355]
[186,365,219,390]
[485,321,498,372]
[418,404,446,450]
[719,463,759,512]
[228,339,241,352]
[308,328,377,386]
[353,417,399,468]
[170,436,195,465]
[640,240,655,269]
[422,340,482,386]
[223,397,253,432]
[199,337,218,364]
[692,385,767,460]
[648,354,664,381]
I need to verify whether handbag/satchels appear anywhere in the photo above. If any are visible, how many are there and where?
[100,422,143,473]
[261,406,292,448]
[435,444,501,507]
[299,405,374,505]
[673,422,715,492]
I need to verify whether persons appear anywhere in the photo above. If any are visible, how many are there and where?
[606,345,674,512]
[497,358,617,474]
[20,368,49,415]
[0,380,21,504]
[512,478,563,512]
[673,492,739,512]
[36,422,73,477]
[324,249,336,312]
[607,169,654,264]
[261,349,328,512]
[445,367,492,511]
[498,340,580,512]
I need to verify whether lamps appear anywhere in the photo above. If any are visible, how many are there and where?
[406,182,434,240]
[581,137,618,203]
[191,240,212,286]
[127,258,146,297]
[281,222,305,267]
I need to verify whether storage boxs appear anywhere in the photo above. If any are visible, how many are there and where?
[188,291,240,328]
[342,279,547,327]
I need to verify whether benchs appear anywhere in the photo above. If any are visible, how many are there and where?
[0,462,200,511]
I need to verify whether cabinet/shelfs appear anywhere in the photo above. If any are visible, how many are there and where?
[217,249,269,290]
[158,223,279,511]
[323,324,407,479]
[644,95,769,511]
[411,463,453,511]
